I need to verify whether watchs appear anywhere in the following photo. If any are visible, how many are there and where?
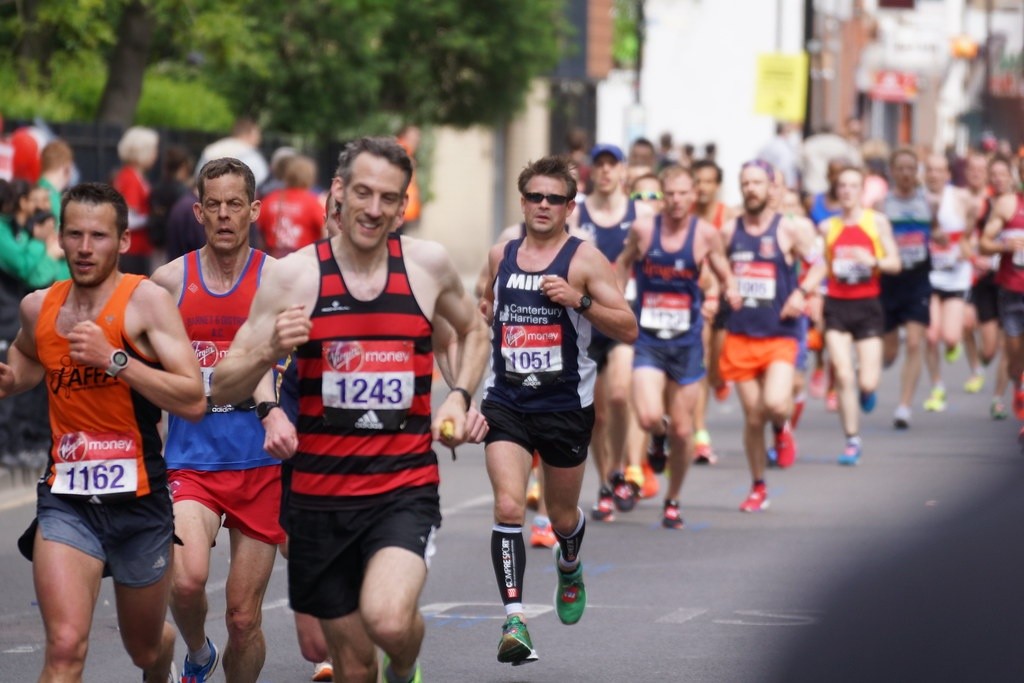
[574,295,591,314]
[106,349,129,380]
[254,401,280,422]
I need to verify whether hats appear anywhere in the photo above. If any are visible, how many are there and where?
[591,143,622,162]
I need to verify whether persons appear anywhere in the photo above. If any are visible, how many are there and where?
[476,115,1024,551]
[0,111,491,683]
[478,155,636,667]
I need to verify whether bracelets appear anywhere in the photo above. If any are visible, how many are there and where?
[873,258,879,269]
[794,286,807,299]
[448,387,471,412]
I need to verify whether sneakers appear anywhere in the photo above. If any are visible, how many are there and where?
[177,635,218,683]
[496,616,539,667]
[554,541,587,626]
[530,514,559,549]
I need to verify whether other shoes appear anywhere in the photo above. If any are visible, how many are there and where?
[825,387,838,412]
[626,461,658,499]
[772,424,795,468]
[964,375,983,393]
[990,400,1009,420]
[923,387,946,413]
[893,406,911,428]
[945,344,959,363]
[810,367,826,399]
[838,440,862,465]
[646,415,671,475]
[591,485,614,520]
[663,498,683,529]
[860,392,877,412]
[693,429,711,464]
[312,657,336,682]
[382,651,421,683]
[526,481,541,509]
[607,472,640,512]
[1011,374,1024,442]
[739,480,770,512]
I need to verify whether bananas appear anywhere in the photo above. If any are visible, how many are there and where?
[440,420,455,439]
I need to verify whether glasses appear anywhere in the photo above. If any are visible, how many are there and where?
[629,191,664,201]
[523,192,570,205]
